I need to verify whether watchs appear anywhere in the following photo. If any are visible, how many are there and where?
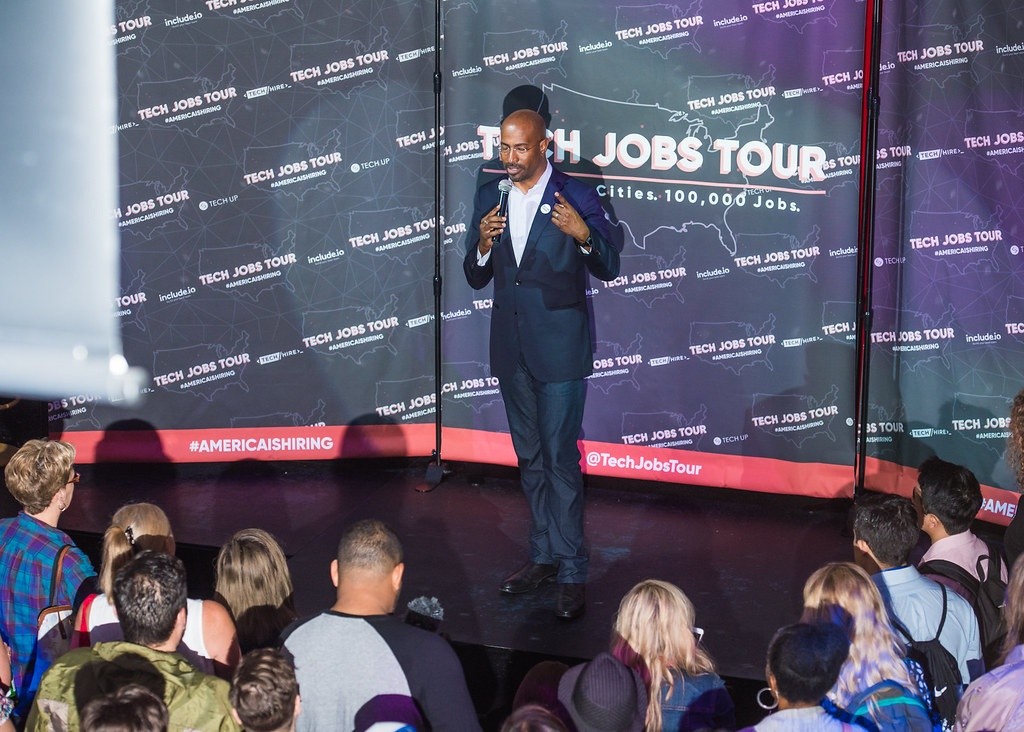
[583,235,592,247]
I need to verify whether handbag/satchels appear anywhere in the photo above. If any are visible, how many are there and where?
[29,545,77,691]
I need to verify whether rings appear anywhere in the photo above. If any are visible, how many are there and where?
[481,218,488,224]
[556,214,559,219]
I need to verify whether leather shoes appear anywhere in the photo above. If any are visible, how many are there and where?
[499,559,558,594]
[556,579,591,619]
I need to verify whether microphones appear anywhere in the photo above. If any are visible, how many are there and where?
[492,180,512,244]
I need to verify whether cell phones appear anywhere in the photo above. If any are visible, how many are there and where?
[691,627,704,648]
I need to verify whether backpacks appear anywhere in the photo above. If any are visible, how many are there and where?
[836,668,930,731]
[917,540,1011,663]
[881,580,965,726]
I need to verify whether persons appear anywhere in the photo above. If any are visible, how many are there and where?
[463,110,621,620]
[1,390,1024,732]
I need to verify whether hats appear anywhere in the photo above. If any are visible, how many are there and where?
[557,653,646,732]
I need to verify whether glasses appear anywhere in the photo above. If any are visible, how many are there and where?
[58,472,80,493]
[691,626,704,647]
